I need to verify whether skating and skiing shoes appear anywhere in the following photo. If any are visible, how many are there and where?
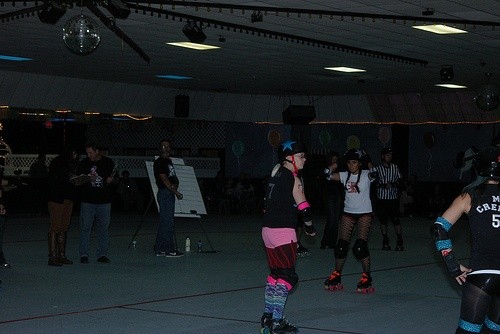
[324,270,343,289]
[262,312,298,334]
[295,246,311,259]
[356,272,375,294]
[382,236,391,250]
[395,237,405,250]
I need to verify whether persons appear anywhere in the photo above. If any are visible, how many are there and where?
[291,167,312,259]
[260,140,317,332]
[44,143,81,266]
[119,170,134,196]
[75,142,121,263]
[322,149,380,289]
[154,141,185,258]
[27,152,49,178]
[321,153,345,249]
[370,147,407,251]
[212,170,268,205]
[432,147,500,334]
[0,169,11,268]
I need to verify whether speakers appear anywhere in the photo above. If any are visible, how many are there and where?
[102,0,131,19]
[39,2,66,25]
[282,105,316,125]
[174,95,190,117]
[182,23,208,44]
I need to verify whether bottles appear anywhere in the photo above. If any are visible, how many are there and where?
[185,237,191,252]
[198,240,202,253]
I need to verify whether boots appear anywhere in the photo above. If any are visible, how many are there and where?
[48,232,73,265]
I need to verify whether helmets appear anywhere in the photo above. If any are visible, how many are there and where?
[473,145,500,179]
[278,139,307,157]
[343,148,366,161]
[380,145,395,154]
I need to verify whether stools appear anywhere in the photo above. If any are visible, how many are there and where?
[217,198,235,217]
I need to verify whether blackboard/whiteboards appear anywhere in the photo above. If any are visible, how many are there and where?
[145,160,208,215]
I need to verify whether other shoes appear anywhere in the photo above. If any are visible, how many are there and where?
[97,256,109,262]
[320,244,335,250]
[80,257,88,263]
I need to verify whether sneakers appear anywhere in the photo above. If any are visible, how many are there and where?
[156,249,185,257]
